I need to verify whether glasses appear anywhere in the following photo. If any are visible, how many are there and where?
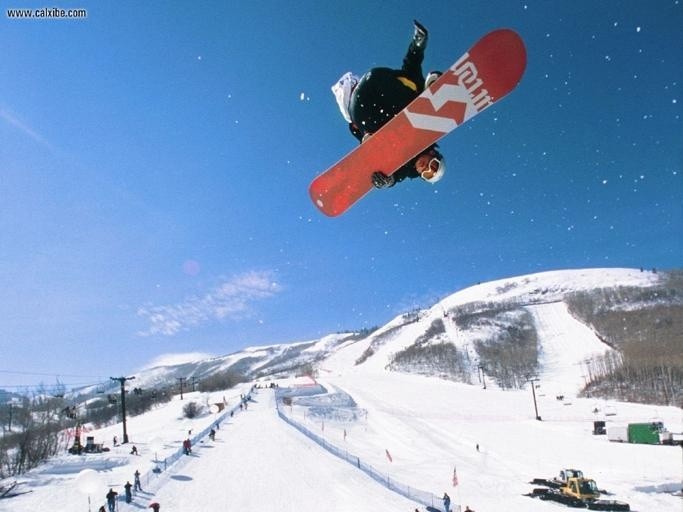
[421,157,440,181]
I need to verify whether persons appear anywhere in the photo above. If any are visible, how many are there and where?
[187,437,192,452]
[442,491,450,511]
[124,480,131,502]
[183,439,188,455]
[328,19,446,190]
[131,445,138,455]
[105,488,118,511]
[133,468,141,491]
[209,428,215,440]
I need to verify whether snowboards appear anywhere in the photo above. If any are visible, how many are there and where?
[309,30,526,217]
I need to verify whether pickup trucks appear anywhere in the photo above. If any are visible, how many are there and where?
[548,468,600,504]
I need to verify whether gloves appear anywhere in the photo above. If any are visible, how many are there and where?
[371,172,391,189]
[412,19,428,48]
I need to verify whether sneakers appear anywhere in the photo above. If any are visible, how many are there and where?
[425,71,442,88]
[362,132,374,142]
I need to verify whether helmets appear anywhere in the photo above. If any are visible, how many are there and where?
[421,153,447,185]
